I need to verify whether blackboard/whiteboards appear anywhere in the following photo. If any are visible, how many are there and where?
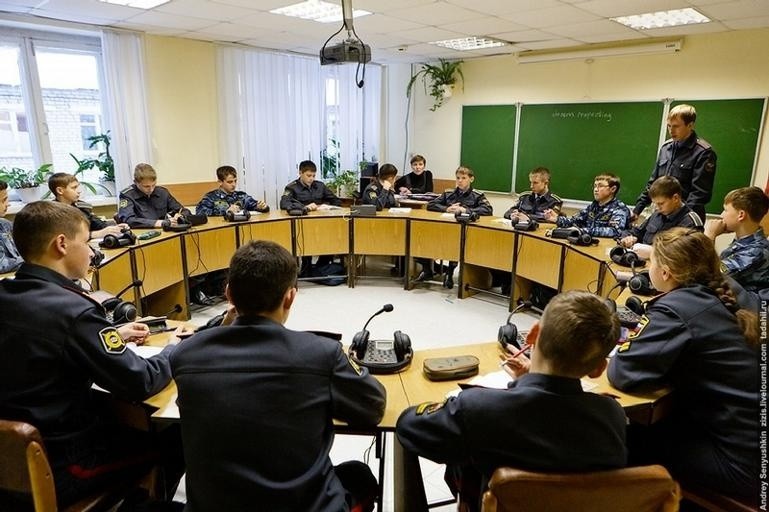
[460,96,767,221]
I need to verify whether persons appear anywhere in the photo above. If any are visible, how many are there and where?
[629,103,718,225]
[1,155,769,511]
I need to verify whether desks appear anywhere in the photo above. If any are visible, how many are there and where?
[1,178,769,512]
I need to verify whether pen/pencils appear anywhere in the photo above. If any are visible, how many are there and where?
[150,326,178,335]
[498,345,531,367]
[178,207,183,216]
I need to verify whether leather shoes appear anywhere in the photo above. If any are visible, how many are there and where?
[193,290,215,306]
[414,271,433,281]
[444,272,454,289]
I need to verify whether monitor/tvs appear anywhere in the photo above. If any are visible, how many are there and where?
[360,161,378,176]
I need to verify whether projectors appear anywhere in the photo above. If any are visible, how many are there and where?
[320,44,371,66]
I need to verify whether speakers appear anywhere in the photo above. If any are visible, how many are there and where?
[360,177,376,196]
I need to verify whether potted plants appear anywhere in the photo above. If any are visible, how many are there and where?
[0,162,53,203]
[76,128,117,197]
[403,52,467,112]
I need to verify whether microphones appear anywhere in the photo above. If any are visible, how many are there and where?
[363,304,393,330]
[116,280,143,298]
[631,258,639,276]
[606,281,626,298]
[459,203,472,212]
[507,301,532,324]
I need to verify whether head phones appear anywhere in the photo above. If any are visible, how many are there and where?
[348,330,413,373]
[455,209,646,267]
[89,203,309,267]
[100,297,137,325]
[498,323,531,358]
[629,269,663,295]
[605,296,645,329]
